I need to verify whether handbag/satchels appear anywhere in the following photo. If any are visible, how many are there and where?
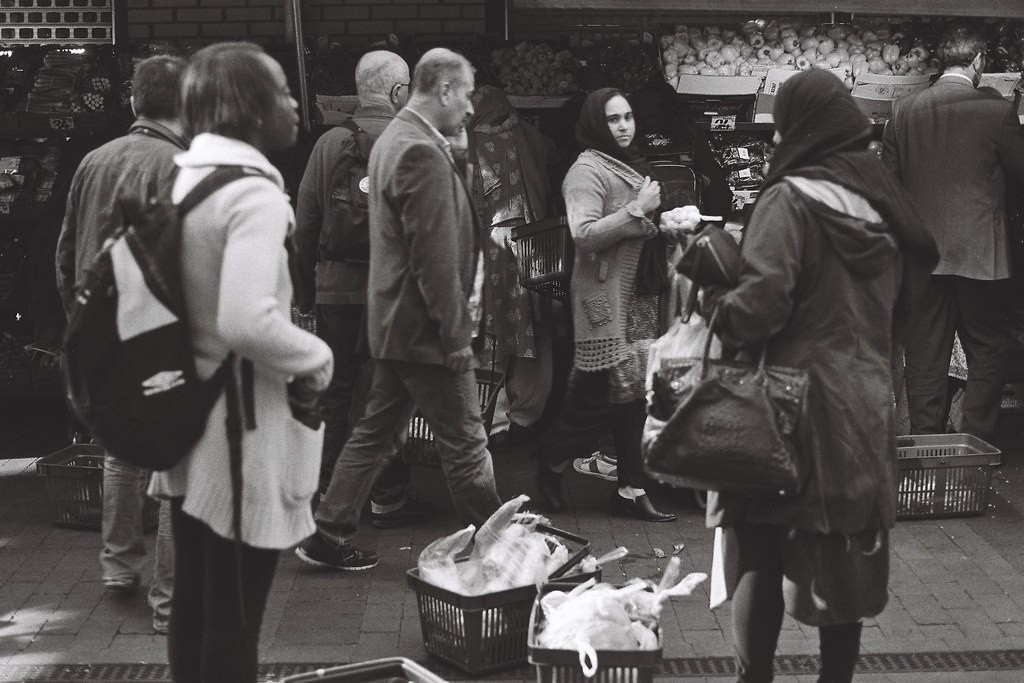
[672,223,739,322]
[644,296,810,499]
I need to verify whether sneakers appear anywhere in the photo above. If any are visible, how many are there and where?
[573,451,620,482]
[151,615,170,634]
[294,533,379,570]
[102,573,140,590]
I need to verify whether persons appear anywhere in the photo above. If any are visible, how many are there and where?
[55,56,192,638]
[287,48,441,532]
[141,38,335,683]
[881,23,1024,467]
[292,46,504,573]
[692,72,909,683]
[526,89,680,526]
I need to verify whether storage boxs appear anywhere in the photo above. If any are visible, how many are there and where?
[402,368,506,468]
[406,517,603,676]
[897,433,1000,521]
[527,582,663,683]
[36,444,160,534]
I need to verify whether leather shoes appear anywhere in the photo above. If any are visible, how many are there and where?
[532,485,567,513]
[613,491,678,521]
[373,499,434,528]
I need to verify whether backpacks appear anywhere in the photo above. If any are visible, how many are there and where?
[319,118,383,260]
[65,165,289,472]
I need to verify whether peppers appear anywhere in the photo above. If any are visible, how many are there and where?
[661,17,1024,91]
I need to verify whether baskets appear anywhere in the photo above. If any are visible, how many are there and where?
[388,330,507,466]
[406,518,600,675]
[895,433,1001,520]
[528,581,663,683]
[36,444,174,534]
[509,215,573,302]
